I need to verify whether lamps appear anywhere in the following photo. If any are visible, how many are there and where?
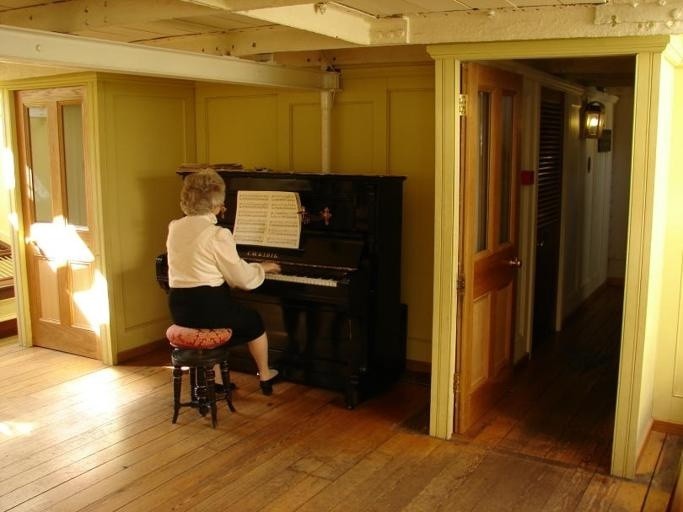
[580,101,605,139]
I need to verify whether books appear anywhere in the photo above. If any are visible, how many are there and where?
[231,189,303,249]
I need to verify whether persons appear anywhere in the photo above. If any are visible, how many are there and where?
[164,168,296,396]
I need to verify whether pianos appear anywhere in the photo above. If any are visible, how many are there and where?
[156,163,406,409]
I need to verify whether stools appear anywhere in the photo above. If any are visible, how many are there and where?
[166,324,237,429]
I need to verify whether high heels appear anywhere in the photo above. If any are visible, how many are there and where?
[216,382,235,395]
[261,367,286,394]
